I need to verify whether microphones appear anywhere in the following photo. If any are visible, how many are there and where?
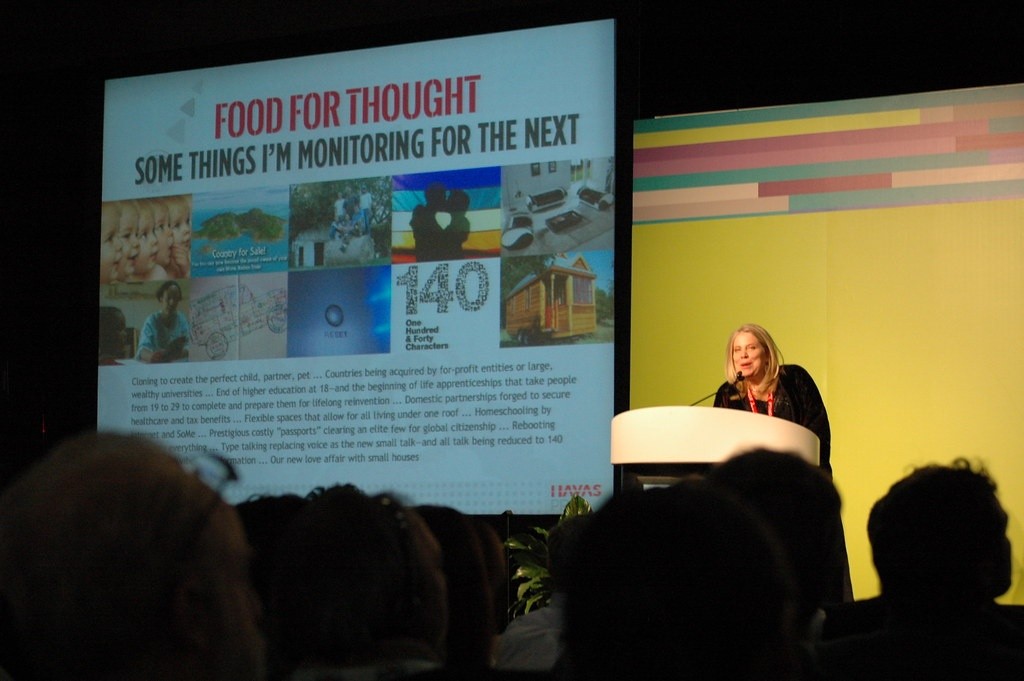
[689,371,751,411]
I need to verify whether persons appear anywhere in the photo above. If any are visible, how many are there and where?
[823,458,1024,681]
[97,194,193,366]
[712,323,832,484]
[1,431,271,681]
[235,446,855,680]
[328,183,372,253]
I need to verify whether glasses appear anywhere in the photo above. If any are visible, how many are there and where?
[149,451,237,597]
